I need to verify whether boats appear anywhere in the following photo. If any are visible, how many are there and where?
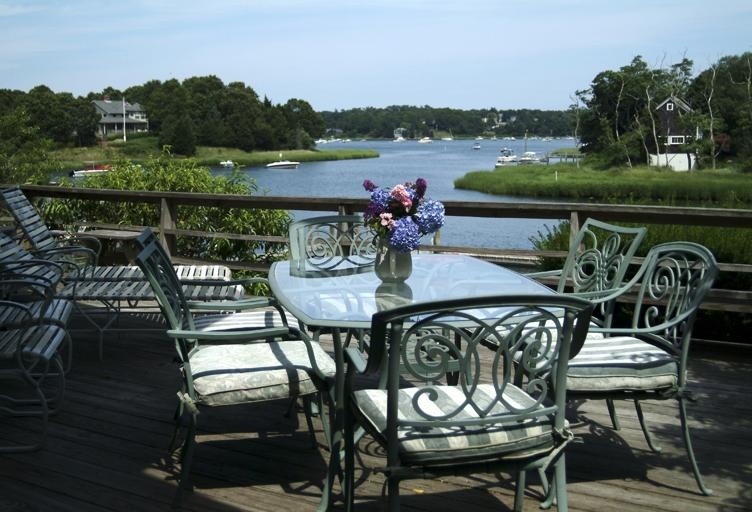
[266,160,299,169]
[221,160,234,168]
[314,134,574,168]
[72,164,144,177]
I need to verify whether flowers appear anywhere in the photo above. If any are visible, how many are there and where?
[362,178,447,279]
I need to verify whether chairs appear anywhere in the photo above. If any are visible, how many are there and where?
[505,246,717,496]
[140,245,333,497]
[0,234,247,359]
[1,281,66,454]
[449,219,650,432]
[342,297,594,509]
[136,231,318,416]
[3,186,231,320]
[2,260,74,402]
[289,214,419,368]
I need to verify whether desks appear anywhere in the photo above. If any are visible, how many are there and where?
[82,230,146,267]
